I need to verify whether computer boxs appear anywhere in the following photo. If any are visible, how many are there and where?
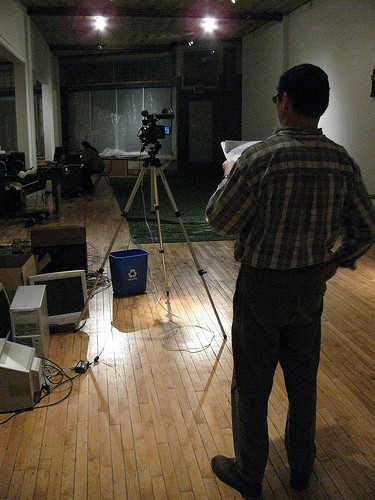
[9,285,50,361]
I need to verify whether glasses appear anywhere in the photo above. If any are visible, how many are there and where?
[272,93,282,104]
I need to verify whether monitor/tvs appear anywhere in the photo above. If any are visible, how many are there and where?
[0,283,12,340]
[29,270,90,331]
[0,339,42,413]
[54,147,63,164]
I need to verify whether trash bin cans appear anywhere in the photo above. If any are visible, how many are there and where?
[108,248,150,296]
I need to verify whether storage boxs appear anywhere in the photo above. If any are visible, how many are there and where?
[0,253,35,306]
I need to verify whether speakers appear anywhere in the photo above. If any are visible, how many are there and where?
[10,152,26,174]
[0,154,7,173]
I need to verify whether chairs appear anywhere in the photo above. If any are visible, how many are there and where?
[95,159,115,188]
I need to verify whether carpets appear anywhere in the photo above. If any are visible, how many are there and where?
[109,175,241,244]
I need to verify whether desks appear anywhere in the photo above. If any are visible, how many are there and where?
[0,167,63,224]
[53,156,85,197]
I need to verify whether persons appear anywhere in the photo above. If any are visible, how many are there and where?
[79,141,105,194]
[205,63,375,499]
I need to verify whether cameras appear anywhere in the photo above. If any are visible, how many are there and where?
[138,108,175,159]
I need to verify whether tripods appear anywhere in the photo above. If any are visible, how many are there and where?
[75,152,228,340]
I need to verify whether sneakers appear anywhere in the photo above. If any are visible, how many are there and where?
[211,455,263,499]
[289,441,316,490]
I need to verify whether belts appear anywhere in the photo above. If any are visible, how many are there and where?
[284,262,330,275]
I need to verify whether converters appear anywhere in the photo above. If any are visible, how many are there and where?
[75,360,89,373]
